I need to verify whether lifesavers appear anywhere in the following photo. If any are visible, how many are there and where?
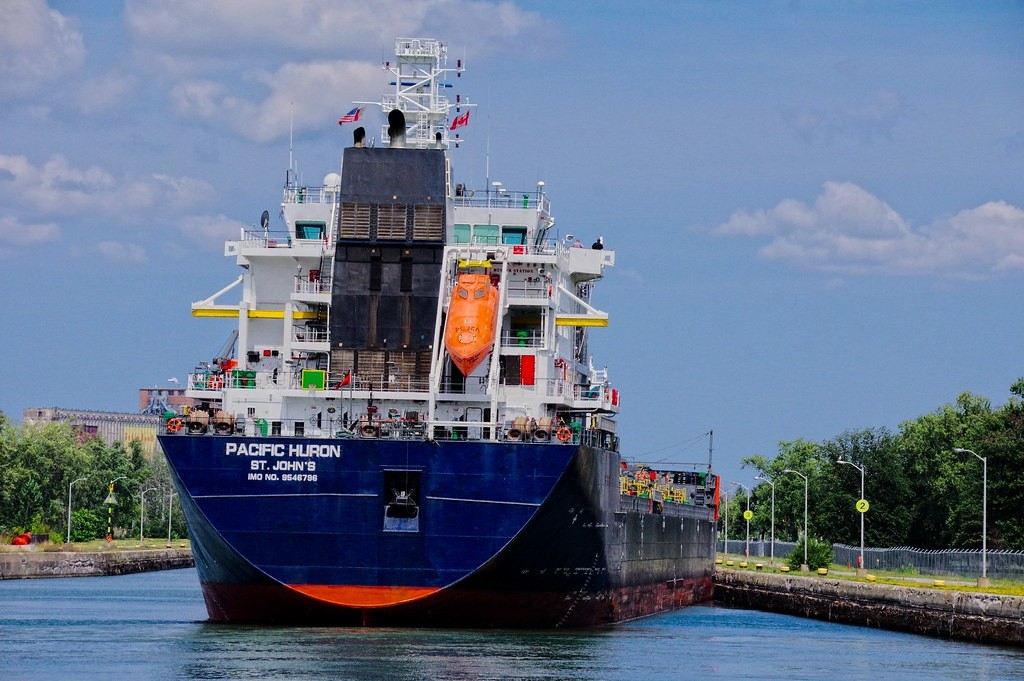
[208,376,223,387]
[167,419,182,432]
[557,428,571,441]
[107,536,112,543]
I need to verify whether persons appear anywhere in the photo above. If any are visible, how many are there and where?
[858,555,862,567]
[744,547,747,556]
[572,240,584,248]
[647,483,654,499]
[456,184,463,196]
[664,473,668,481]
[592,239,603,250]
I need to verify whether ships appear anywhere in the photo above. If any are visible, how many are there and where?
[155,37,720,626]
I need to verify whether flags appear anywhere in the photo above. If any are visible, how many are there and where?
[339,106,365,126]
[335,370,351,389]
[450,115,458,130]
[458,110,469,125]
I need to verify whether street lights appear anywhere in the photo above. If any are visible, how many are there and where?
[954,448,991,589]
[754,476,775,564]
[731,482,750,557]
[67,476,87,545]
[837,461,867,579]
[140,488,157,547]
[784,469,809,574]
